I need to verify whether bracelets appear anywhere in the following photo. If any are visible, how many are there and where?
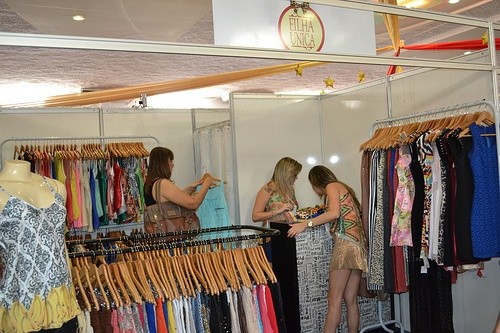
[271,210,275,217]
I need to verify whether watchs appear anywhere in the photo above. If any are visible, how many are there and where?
[306,219,313,228]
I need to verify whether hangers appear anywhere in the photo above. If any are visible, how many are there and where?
[65,225,278,312]
[13,138,149,161]
[359,100,497,152]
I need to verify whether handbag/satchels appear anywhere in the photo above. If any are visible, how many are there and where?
[259,237,273,263]
[357,278,376,298]
[144,177,200,244]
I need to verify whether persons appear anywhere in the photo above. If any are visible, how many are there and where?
[251,156,370,333]
[0,159,79,333]
[143,146,217,234]
